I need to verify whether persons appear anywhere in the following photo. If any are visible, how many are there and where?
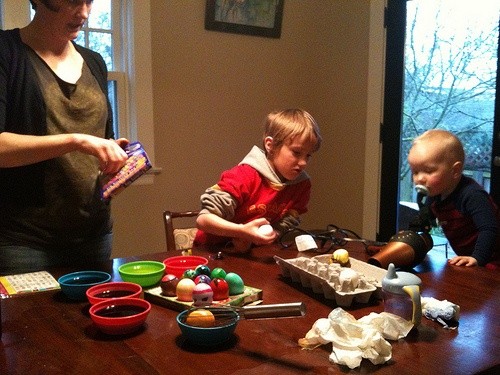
[191,108,322,247]
[408,127,500,266]
[0,0,129,275]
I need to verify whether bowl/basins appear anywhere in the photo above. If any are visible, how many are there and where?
[57,271,112,299]
[176,306,240,342]
[163,256,208,279]
[86,282,144,307]
[118,260,166,287]
[89,298,152,334]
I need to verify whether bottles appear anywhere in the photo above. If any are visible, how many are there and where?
[382,263,422,329]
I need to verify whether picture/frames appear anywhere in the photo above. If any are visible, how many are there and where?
[201,0,288,41]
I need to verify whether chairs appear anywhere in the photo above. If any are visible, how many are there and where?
[155,205,207,252]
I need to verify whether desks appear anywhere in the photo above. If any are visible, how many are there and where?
[0,242,500,375]
